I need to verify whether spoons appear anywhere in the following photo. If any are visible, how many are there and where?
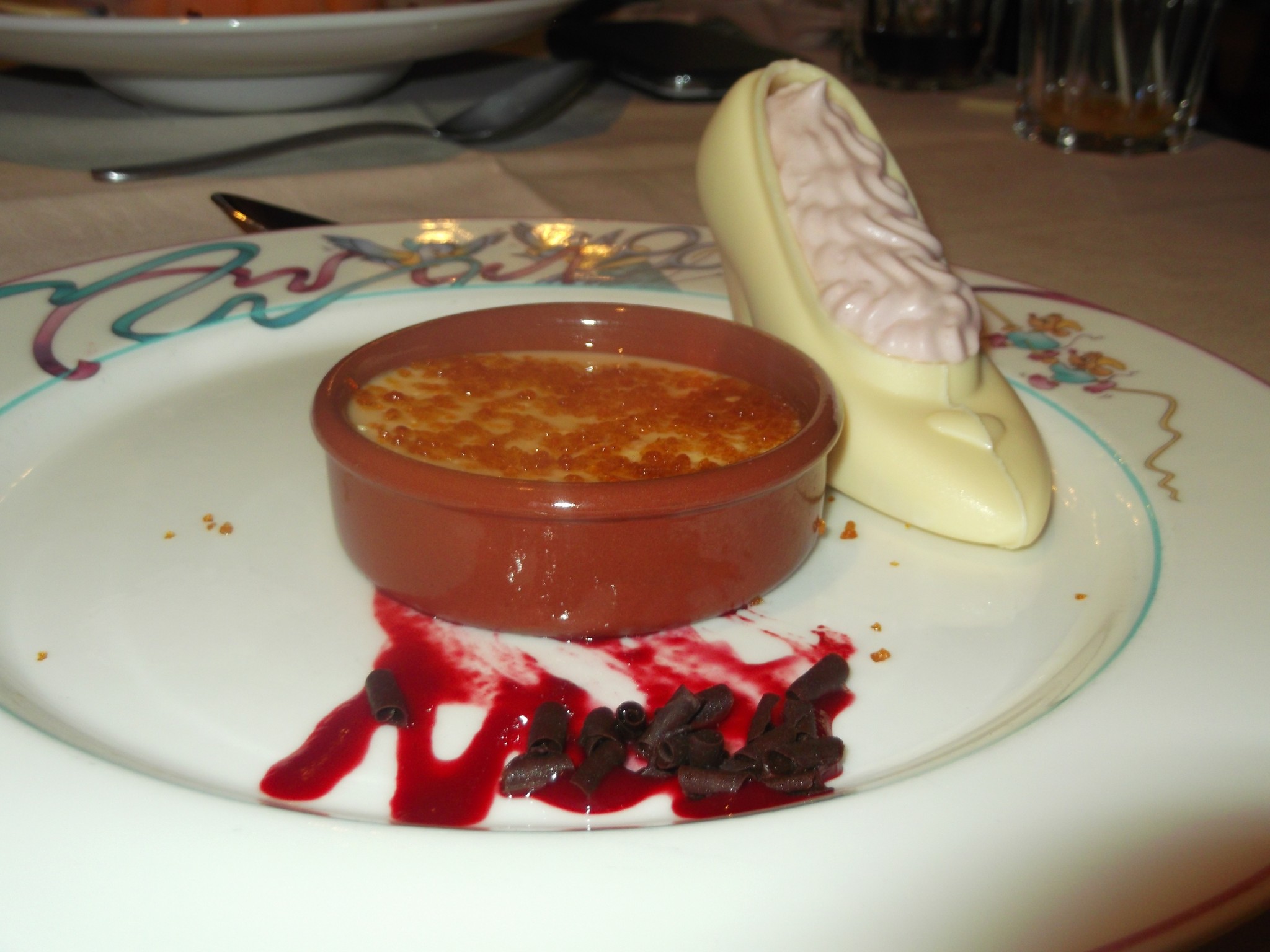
[211,192,340,232]
[91,63,609,181]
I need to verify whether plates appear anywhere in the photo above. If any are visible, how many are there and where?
[2,215,1269,951]
[1,0,573,112]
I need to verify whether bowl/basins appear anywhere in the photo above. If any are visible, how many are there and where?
[309,302,846,640]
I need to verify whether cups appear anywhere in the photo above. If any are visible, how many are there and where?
[838,1,1018,91]
[1015,0,1217,154]
